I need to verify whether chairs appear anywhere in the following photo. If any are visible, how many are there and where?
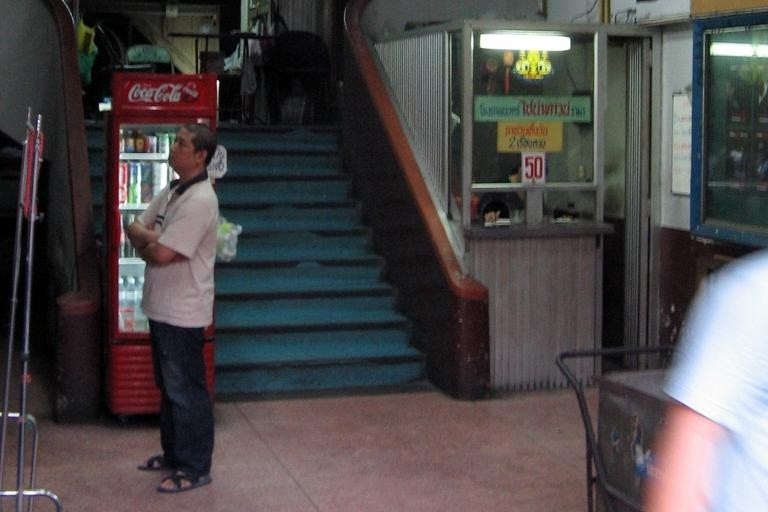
[257,27,332,126]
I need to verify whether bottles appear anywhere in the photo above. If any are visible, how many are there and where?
[119,128,148,153]
[118,276,146,332]
[119,213,141,258]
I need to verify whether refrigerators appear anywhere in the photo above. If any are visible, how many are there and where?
[106,70,222,416]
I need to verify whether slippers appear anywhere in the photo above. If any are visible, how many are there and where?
[137,454,171,470]
[156,471,211,492]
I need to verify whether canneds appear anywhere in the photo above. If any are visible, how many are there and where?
[119,161,152,205]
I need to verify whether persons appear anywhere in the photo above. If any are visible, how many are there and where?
[639,247,768,512]
[125,122,220,494]
[476,161,549,219]
